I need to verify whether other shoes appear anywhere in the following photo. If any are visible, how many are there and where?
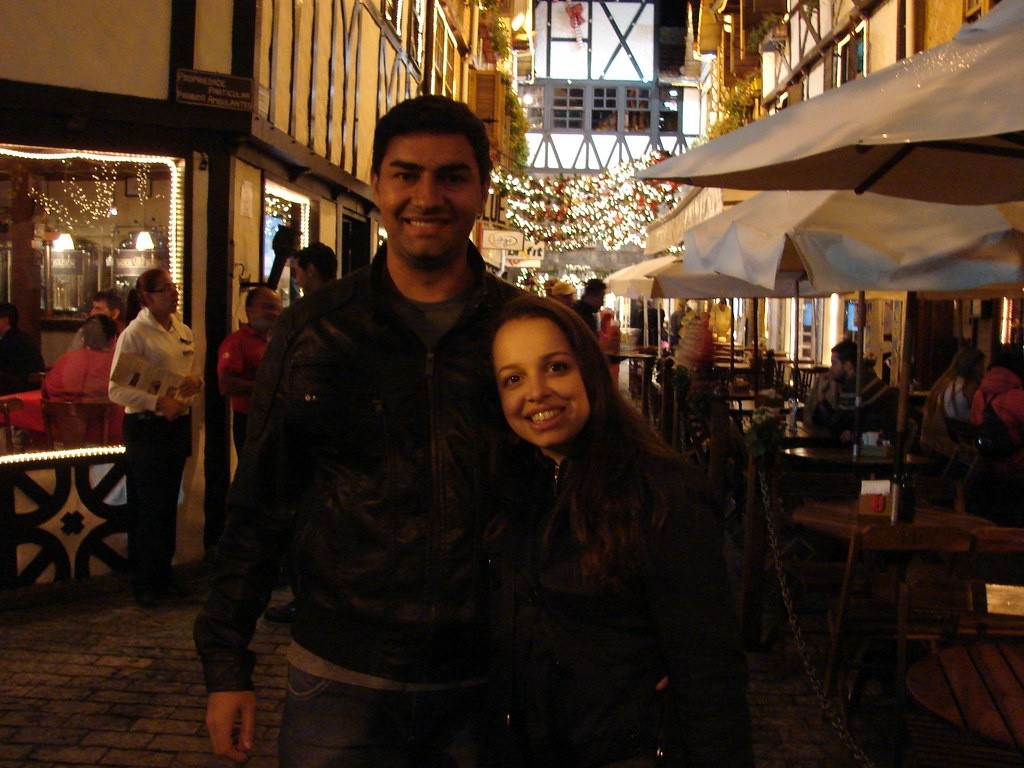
[169,591,199,607]
[138,593,160,610]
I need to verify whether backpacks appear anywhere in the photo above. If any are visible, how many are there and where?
[975,384,1017,460]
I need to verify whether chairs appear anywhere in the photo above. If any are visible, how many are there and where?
[819,524,970,722]
[41,401,111,449]
[958,527,1024,641]
[890,574,1024,768]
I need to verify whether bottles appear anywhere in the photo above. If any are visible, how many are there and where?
[175,384,198,401]
[858,465,917,525]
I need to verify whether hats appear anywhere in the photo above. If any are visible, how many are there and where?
[551,282,577,296]
[87,313,117,340]
[543,280,560,290]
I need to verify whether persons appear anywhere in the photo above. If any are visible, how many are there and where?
[0,302,45,398]
[43,284,135,440]
[919,342,1024,515]
[219,285,280,459]
[293,241,338,297]
[804,340,893,446]
[191,94,532,768]
[543,279,577,306]
[629,298,731,394]
[578,279,605,337]
[107,271,205,607]
[459,294,747,768]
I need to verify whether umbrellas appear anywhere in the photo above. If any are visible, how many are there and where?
[605,0,1024,298]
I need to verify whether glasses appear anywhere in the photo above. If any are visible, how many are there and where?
[152,285,177,295]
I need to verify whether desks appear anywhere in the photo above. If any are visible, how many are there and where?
[711,342,1023,768]
[0,389,127,455]
